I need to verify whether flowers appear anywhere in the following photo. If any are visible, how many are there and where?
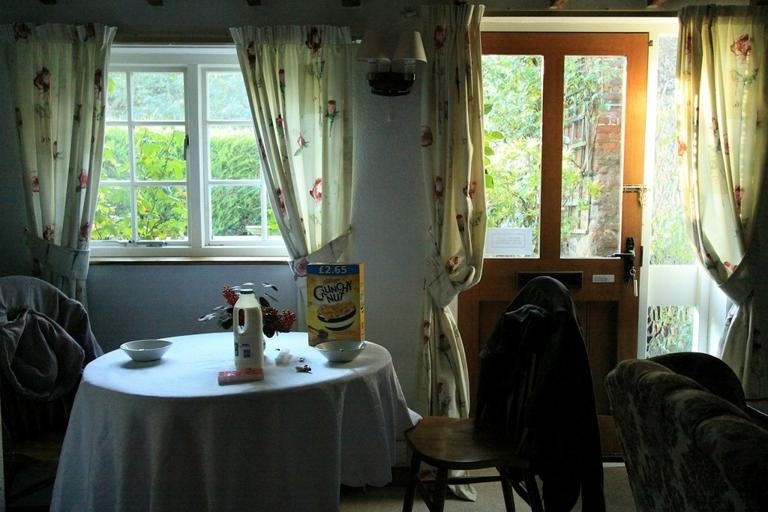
[196,284,298,340]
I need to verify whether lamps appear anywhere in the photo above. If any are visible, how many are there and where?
[353,11,428,96]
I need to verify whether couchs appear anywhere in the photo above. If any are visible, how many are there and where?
[603,351,768,510]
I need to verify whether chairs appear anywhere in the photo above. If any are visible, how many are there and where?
[400,276,579,510]
[0,275,104,512]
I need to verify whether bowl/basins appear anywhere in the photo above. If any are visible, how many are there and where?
[315,341,367,361]
[120,341,171,360]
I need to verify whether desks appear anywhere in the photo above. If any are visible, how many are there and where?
[48,333,422,512]
